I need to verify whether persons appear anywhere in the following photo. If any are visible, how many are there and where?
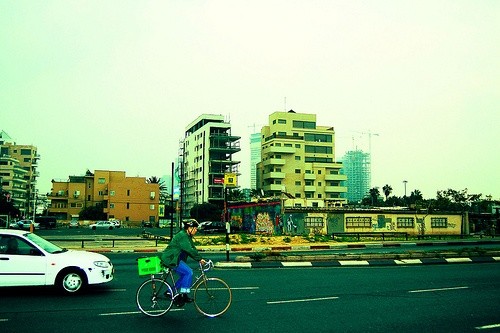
[161,219,205,303]
[141,220,144,229]
[491,225,495,238]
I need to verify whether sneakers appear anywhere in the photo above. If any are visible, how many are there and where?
[163,290,172,299]
[177,293,194,303]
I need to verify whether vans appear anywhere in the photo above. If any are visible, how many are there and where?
[30,217,56,230]
[180,218,199,229]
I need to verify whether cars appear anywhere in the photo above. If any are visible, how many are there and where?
[112,221,121,228]
[161,222,177,227]
[89,221,115,230]
[69,220,79,229]
[0,229,115,296]
[10,220,40,230]
[144,222,154,227]
[197,221,219,232]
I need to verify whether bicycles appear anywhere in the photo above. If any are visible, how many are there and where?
[137,258,232,317]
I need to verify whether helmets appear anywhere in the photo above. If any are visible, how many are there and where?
[182,219,199,227]
